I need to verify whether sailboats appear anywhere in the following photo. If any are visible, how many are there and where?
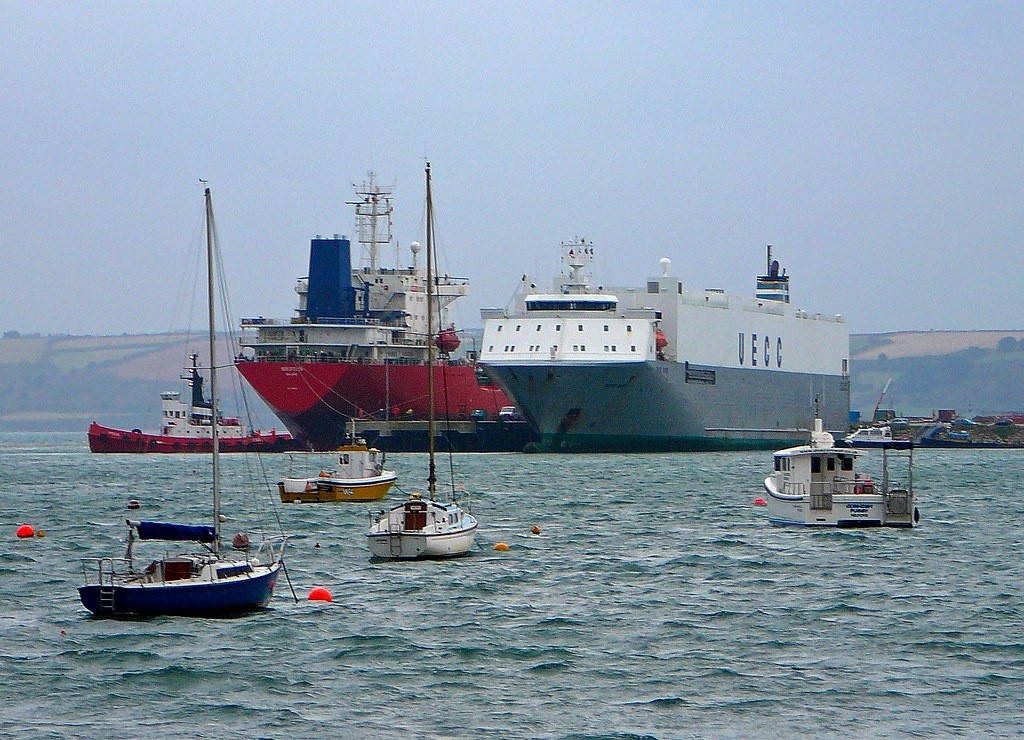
[75,188,292,616]
[357,160,480,556]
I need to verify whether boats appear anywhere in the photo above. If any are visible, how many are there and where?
[88,351,297,454]
[276,414,395,502]
[761,418,923,532]
[844,426,913,449]
[478,232,853,449]
[228,172,520,448]
